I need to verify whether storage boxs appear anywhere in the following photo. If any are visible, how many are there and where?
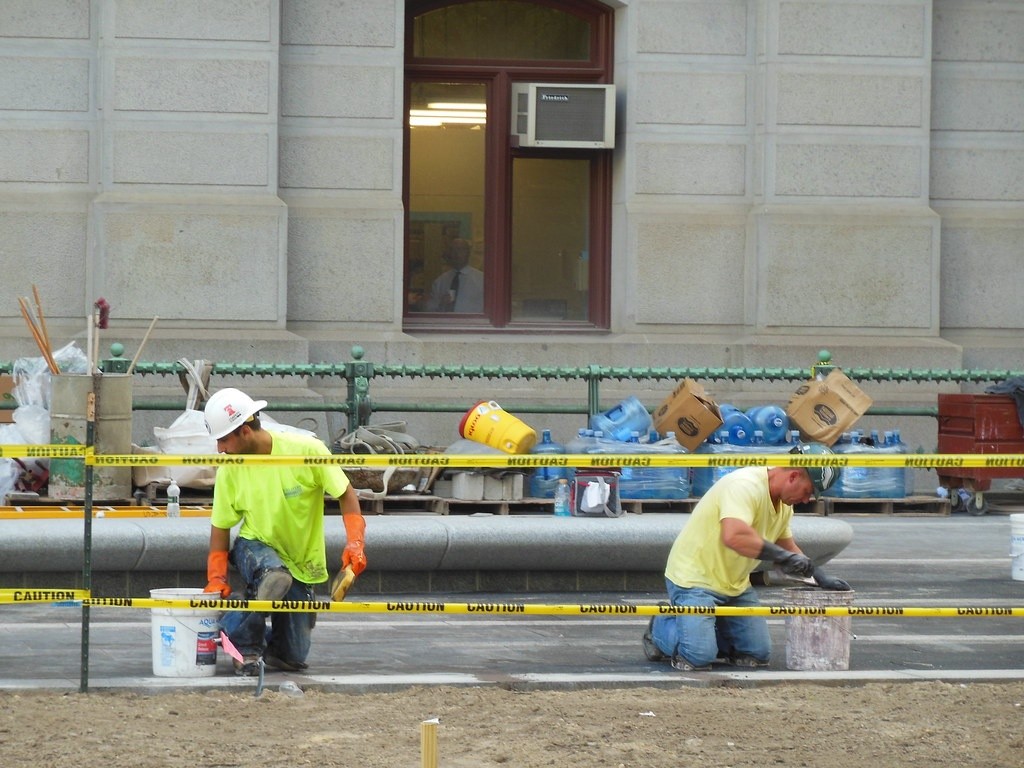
[652,377,723,454]
[784,367,872,448]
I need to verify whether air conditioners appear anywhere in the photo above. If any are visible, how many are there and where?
[511,82,617,150]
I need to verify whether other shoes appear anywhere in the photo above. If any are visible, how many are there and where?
[232,644,267,675]
[642,601,671,662]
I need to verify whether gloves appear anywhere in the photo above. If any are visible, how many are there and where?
[806,558,851,591]
[755,537,816,578]
[340,513,368,578]
[201,550,232,599]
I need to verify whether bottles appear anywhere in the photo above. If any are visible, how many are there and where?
[531,397,917,501]
[167,480,181,518]
[554,478,571,516]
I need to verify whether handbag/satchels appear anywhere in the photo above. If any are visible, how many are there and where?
[568,468,629,518]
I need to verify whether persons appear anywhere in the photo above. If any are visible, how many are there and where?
[201,388,367,676]
[428,238,486,313]
[640,441,852,671]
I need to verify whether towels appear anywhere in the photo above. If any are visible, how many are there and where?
[579,480,610,514]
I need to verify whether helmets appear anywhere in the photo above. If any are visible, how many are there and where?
[789,438,842,504]
[203,387,268,440]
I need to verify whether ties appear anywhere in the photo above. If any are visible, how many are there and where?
[444,272,461,312]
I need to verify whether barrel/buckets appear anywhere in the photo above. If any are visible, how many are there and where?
[781,585,854,671]
[47,372,133,503]
[458,398,536,458]
[149,588,220,678]
[1009,513,1024,582]
